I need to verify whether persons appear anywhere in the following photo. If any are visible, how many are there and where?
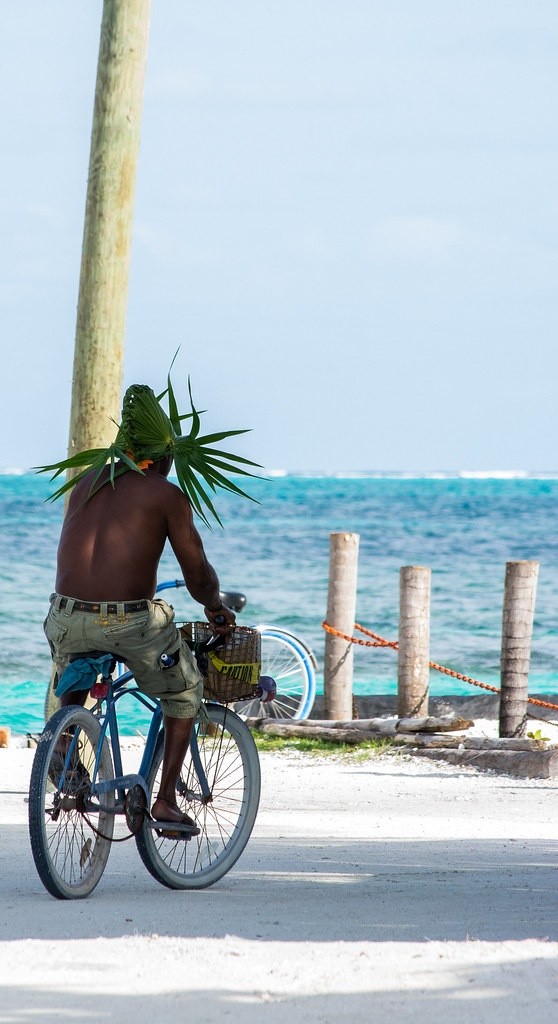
[30,384,236,835]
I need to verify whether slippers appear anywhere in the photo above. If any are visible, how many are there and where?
[49,752,90,797]
[149,813,200,833]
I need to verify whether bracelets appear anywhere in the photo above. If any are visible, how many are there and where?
[207,599,223,612]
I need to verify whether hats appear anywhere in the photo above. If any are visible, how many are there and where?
[30,343,273,531]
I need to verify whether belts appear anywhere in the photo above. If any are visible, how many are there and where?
[60,599,149,614]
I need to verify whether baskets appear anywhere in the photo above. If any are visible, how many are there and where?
[173,621,263,704]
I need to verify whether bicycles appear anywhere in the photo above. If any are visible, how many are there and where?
[114,577,318,733]
[23,613,261,902]
